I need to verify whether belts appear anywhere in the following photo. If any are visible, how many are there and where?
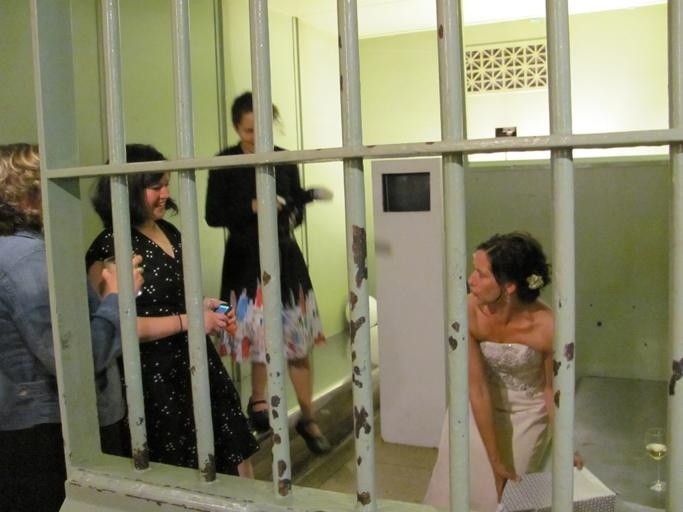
[645,427,669,494]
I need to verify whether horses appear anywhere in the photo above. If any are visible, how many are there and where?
[214,304,232,316]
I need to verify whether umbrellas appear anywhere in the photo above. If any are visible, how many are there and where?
[297,418,330,454]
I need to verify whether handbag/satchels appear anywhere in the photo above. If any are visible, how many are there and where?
[179,314,183,334]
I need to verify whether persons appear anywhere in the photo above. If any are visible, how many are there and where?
[206,90,332,455]
[85,142,260,479]
[0,142,145,512]
[423,229,584,512]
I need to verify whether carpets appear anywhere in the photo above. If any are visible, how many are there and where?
[248,395,268,431]
